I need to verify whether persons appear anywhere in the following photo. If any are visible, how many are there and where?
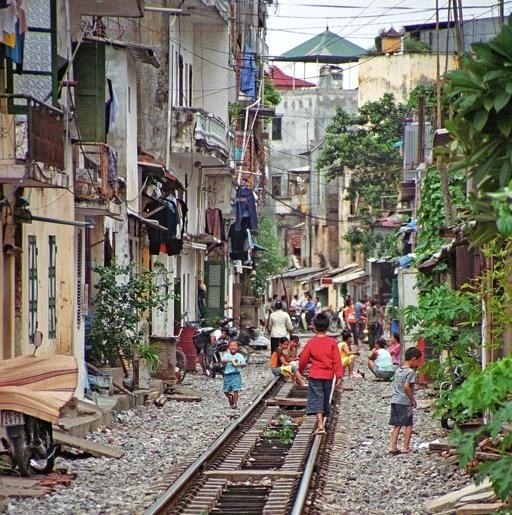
[220,339,248,410]
[294,311,345,436]
[198,326,230,376]
[258,289,404,389]
[389,346,423,454]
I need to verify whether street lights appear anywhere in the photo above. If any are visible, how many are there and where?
[222,89,285,333]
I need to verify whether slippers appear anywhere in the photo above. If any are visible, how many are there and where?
[315,426,326,435]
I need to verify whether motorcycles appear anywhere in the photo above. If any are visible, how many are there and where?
[438,351,481,430]
[184,312,251,380]
[0,329,63,480]
[289,305,316,334]
[327,306,347,333]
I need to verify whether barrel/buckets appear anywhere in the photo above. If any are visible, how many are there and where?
[390,317,400,336]
[417,338,438,384]
[149,336,180,380]
[176,326,201,371]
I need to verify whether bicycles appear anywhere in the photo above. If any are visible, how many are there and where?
[162,323,188,385]
